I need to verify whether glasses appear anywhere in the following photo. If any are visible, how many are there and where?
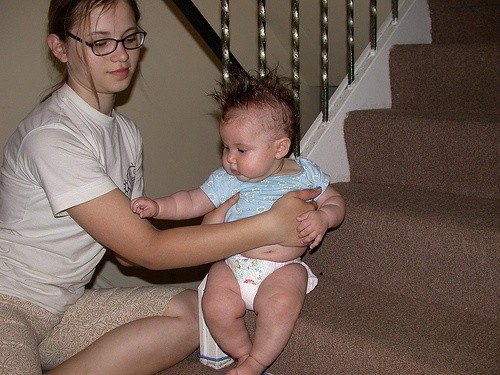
[67,29,147,56]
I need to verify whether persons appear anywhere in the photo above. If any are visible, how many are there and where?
[131,61,345,375]
[0,0,321,375]
